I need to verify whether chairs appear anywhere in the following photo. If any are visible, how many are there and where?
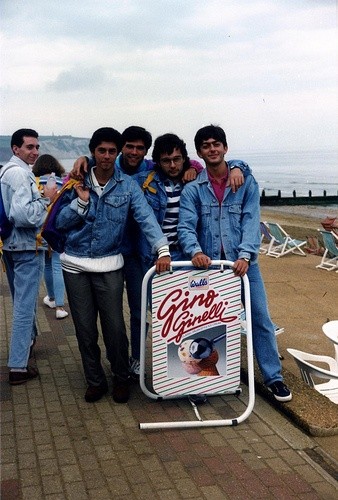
[258,221,307,259]
[315,229,338,272]
[287,348,338,405]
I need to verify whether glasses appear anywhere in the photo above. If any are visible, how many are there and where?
[160,156,184,164]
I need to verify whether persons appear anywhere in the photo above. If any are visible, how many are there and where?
[32,154,68,319]
[71,133,253,404]
[0,128,59,386]
[115,125,204,387]
[55,127,172,404]
[176,125,293,402]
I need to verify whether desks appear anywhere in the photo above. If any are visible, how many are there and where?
[322,320,338,365]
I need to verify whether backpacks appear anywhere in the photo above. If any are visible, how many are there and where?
[0,165,18,240]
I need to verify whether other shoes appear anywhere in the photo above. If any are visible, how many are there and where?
[9,365,39,385]
[189,394,209,404]
[268,380,293,402]
[44,295,56,308]
[129,356,143,374]
[56,309,69,318]
[111,374,132,403]
[84,378,109,403]
[28,330,36,358]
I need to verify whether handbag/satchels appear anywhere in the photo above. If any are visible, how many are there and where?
[41,180,80,253]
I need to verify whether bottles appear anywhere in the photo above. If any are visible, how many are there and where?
[46,172,57,190]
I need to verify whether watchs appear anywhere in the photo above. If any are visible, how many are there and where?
[230,165,239,171]
